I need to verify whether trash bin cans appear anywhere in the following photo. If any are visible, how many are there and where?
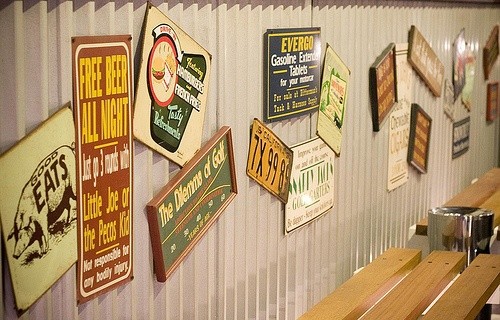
[427,206,494,320]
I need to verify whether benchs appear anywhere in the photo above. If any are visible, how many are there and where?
[299,168,500,320]
